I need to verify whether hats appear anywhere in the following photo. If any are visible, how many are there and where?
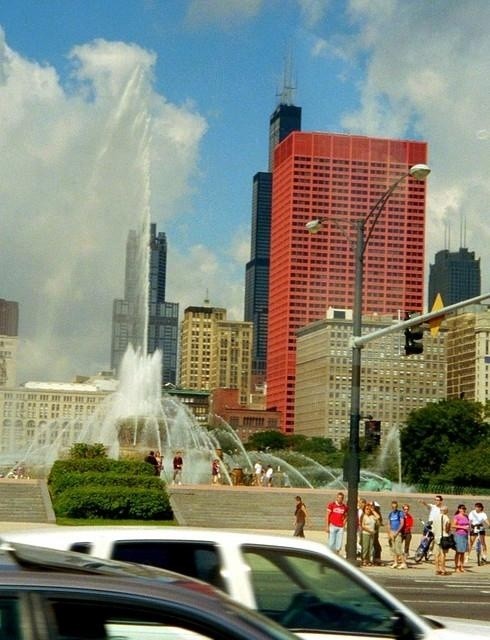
[370,501,381,506]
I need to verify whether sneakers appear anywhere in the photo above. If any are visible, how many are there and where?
[481,556,488,562]
[373,560,385,566]
[455,568,467,573]
[361,561,372,566]
[390,563,408,569]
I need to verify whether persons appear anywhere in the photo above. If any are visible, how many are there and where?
[293,493,490,576]
[145,450,273,487]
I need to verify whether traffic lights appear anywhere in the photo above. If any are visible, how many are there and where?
[365,420,380,447]
[404,310,425,355]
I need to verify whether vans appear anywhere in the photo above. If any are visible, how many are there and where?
[0,524,490,640]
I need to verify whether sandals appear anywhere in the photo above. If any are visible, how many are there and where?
[435,570,450,575]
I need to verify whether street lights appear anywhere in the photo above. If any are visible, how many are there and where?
[305,160,433,569]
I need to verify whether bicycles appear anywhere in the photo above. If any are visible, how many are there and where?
[414,519,433,563]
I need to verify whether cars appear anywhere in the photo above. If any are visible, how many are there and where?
[0,540,295,639]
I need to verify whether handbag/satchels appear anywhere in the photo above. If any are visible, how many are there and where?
[440,534,455,549]
